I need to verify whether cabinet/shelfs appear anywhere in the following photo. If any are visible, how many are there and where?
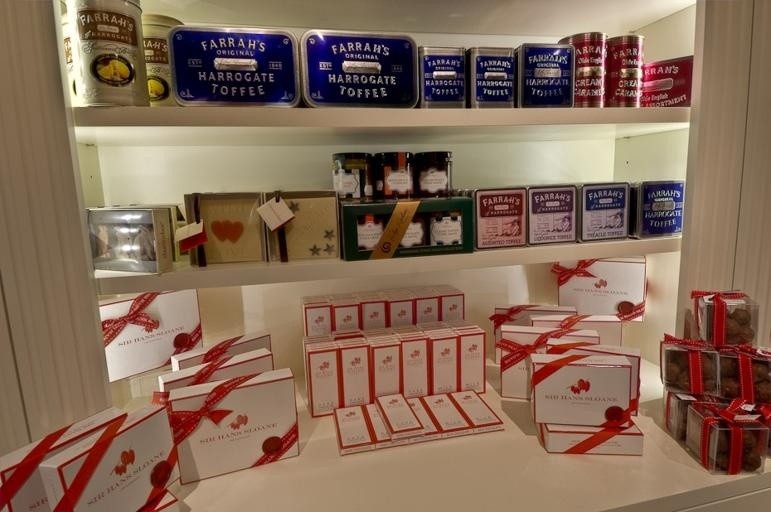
[0,0,771,512]
